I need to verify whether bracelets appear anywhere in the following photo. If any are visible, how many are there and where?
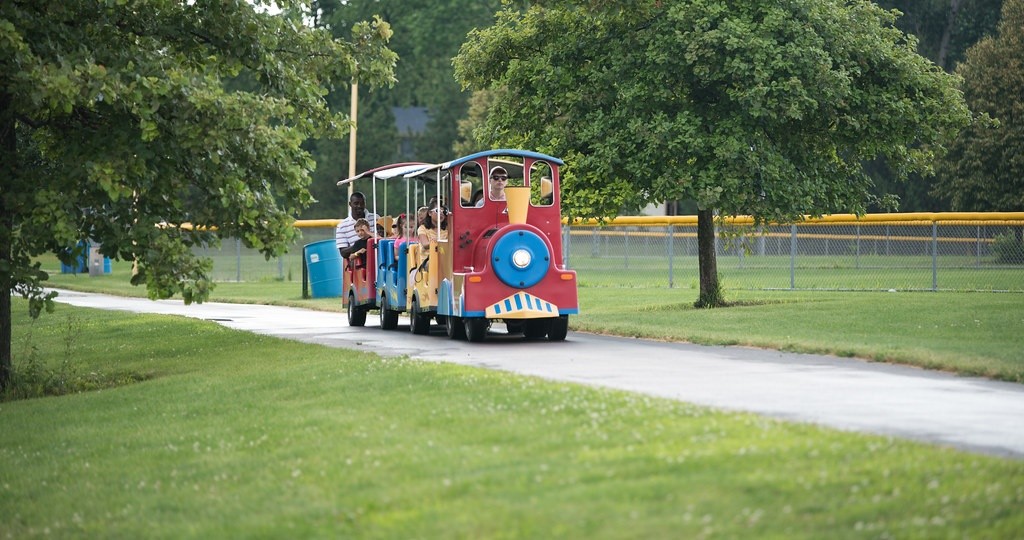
[355,252,358,256]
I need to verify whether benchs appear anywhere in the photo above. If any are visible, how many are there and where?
[342,238,435,289]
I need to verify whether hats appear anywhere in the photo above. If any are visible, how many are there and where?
[376,216,393,235]
[489,166,507,177]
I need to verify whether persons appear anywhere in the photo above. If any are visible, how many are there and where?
[474,165,507,207]
[417,199,447,271]
[349,219,381,269]
[377,216,398,238]
[472,189,483,206]
[336,192,380,269]
[430,195,442,203]
[394,214,419,261]
[417,206,428,224]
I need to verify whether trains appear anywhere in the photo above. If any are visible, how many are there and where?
[336,147,579,341]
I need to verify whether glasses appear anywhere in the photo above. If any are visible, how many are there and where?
[490,176,507,181]
[392,225,397,228]
[430,208,447,215]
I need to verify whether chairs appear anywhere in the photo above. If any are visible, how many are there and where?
[469,187,483,206]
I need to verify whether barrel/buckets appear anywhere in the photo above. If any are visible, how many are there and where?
[304,239,343,298]
